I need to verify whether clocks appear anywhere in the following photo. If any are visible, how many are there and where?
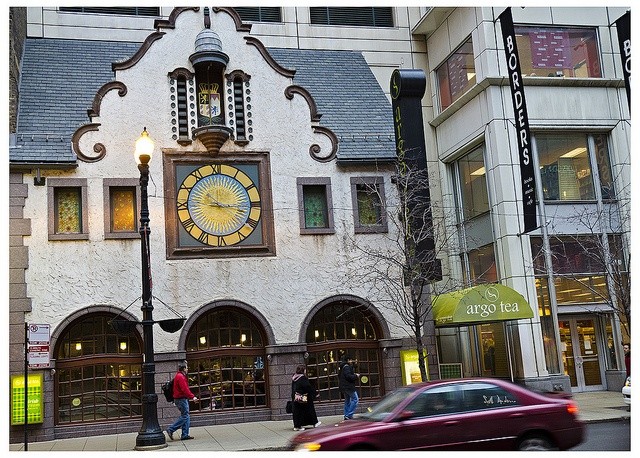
[177,163,260,246]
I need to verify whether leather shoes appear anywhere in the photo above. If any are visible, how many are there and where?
[181,436,194,440]
[167,430,174,440]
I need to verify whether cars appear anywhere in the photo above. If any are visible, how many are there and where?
[291,375,587,451]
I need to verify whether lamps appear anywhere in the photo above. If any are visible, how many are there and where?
[315,330,319,337]
[76,342,81,349]
[240,333,247,342]
[200,335,207,344]
[120,341,126,349]
[352,328,356,334]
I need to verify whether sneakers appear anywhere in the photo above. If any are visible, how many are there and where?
[293,427,305,431]
[314,420,321,428]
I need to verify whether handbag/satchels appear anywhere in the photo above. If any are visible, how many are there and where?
[286,401,293,413]
[162,378,174,404]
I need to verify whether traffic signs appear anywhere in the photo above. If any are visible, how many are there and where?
[27,323,52,341]
[24,344,54,371]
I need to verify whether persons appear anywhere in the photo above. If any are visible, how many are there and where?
[340,355,362,421]
[292,364,320,431]
[623,342,630,385]
[167,364,197,441]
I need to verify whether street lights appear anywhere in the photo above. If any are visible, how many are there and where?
[108,123,186,449]
